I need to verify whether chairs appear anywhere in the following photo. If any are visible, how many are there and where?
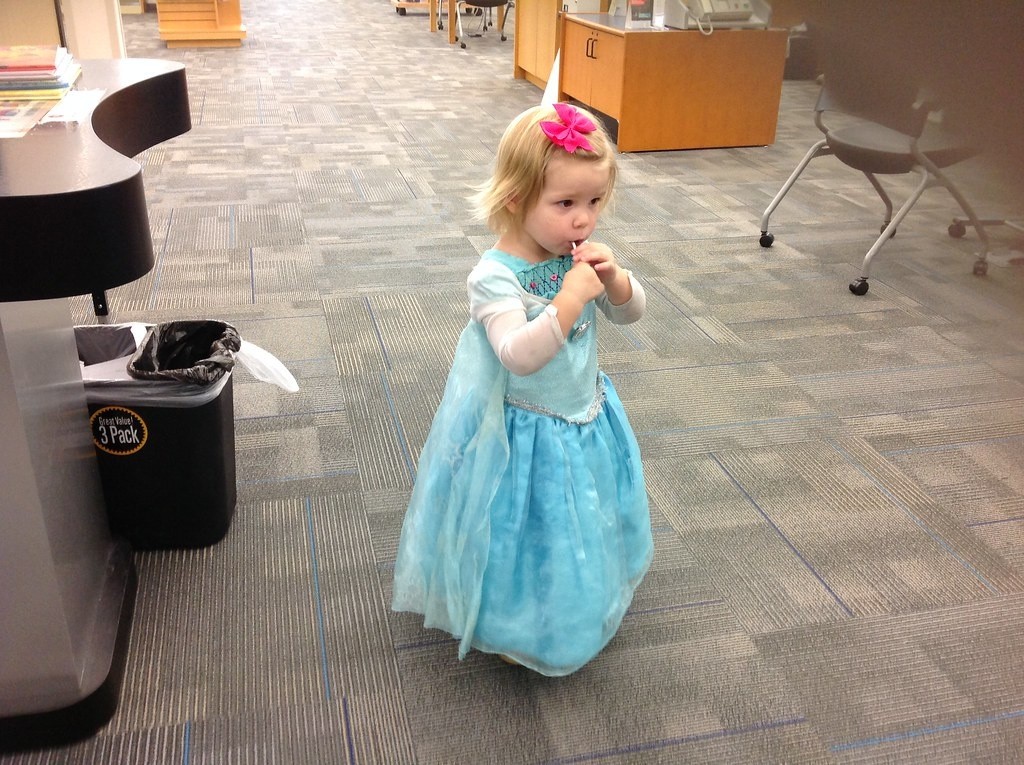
[759,41,992,293]
[455,0,513,48]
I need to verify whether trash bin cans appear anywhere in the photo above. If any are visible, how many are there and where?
[73,318,239,550]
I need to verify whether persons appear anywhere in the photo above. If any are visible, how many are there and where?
[389,102,654,678]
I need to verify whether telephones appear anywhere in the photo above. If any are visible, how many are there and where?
[695,0,754,21]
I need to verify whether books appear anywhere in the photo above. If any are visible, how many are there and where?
[0,45,83,101]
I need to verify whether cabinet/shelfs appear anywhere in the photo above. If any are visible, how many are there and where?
[513,0,790,153]
